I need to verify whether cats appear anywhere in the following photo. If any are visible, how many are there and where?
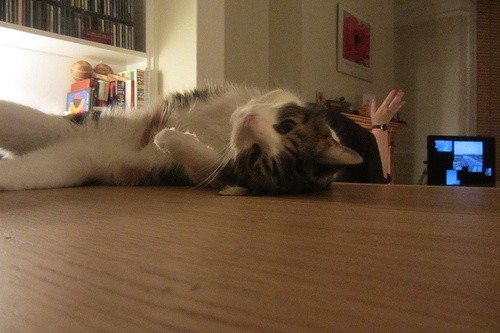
[0,80,364,195]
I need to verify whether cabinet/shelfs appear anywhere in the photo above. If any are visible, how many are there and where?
[0,0,148,57]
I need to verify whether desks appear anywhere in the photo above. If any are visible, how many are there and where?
[337,112,406,185]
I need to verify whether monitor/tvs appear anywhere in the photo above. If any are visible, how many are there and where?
[426,135,496,188]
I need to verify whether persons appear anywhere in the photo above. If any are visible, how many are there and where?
[324,88,407,184]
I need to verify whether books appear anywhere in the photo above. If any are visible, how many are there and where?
[0,0,145,115]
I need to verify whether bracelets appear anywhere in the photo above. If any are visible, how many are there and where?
[373,124,388,131]
